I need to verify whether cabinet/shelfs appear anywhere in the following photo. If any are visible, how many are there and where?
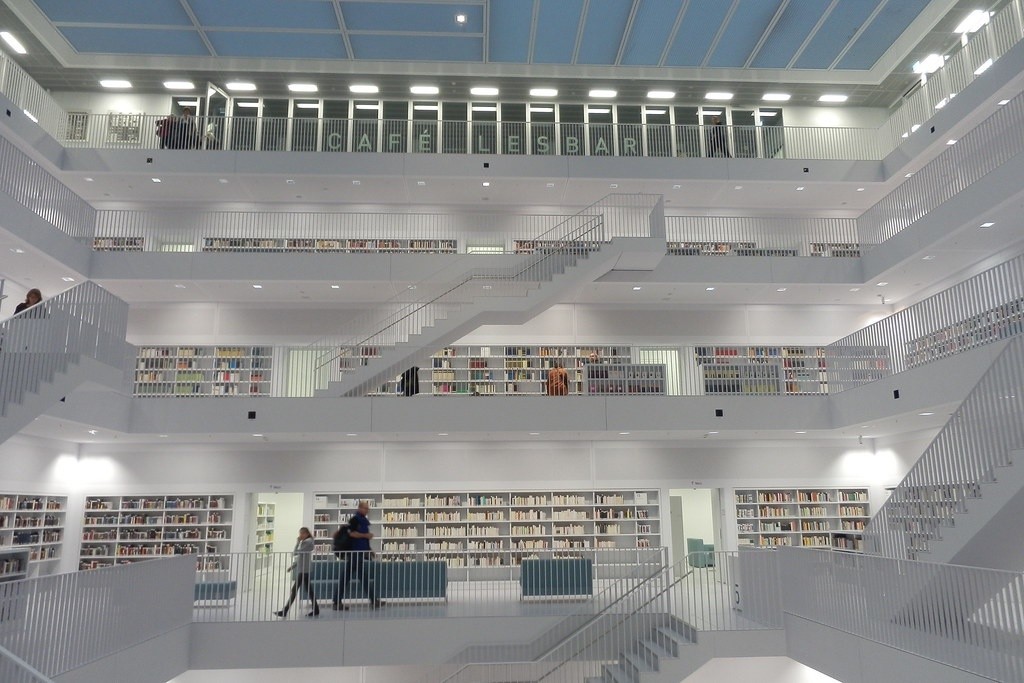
[0,479,980,587]
[667,240,798,258]
[133,345,275,396]
[809,242,860,257]
[336,297,1024,394]
[93,237,147,252]
[515,240,609,255]
[201,237,458,253]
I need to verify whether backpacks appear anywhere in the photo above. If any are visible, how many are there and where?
[333,516,359,559]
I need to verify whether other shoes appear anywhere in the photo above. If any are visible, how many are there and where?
[332,602,349,610]
[372,600,386,610]
[307,610,320,616]
[274,611,287,618]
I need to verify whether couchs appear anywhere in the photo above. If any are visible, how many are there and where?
[686,538,715,567]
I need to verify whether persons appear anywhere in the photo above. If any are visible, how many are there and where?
[13,289,47,319]
[547,361,568,396]
[589,353,600,365]
[400,366,420,397]
[707,116,732,158]
[332,502,385,610]
[274,527,320,619]
[155,107,221,150]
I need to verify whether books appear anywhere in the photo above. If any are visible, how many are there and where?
[95,236,609,254]
[340,346,661,394]
[667,242,861,258]
[0,496,62,575]
[736,491,867,550]
[469,494,650,566]
[79,498,226,572]
[313,496,467,567]
[255,506,274,559]
[887,486,975,560]
[693,300,1024,397]
[136,348,265,394]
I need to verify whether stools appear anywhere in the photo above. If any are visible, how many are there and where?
[193,581,235,603]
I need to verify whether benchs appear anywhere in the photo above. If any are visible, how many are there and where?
[298,559,448,602]
[518,558,593,601]
